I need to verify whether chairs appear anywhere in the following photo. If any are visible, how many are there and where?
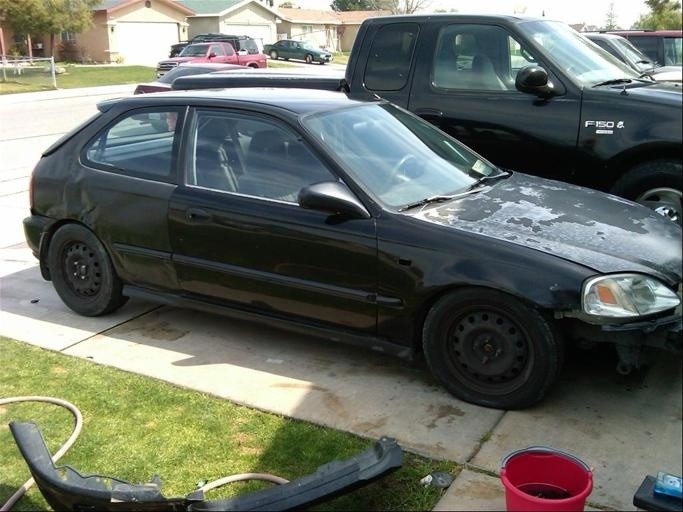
[467,52,506,91]
[238,127,307,206]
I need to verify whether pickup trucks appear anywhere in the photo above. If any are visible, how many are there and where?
[169,11,682,226]
[154,42,268,80]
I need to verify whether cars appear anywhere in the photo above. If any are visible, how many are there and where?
[262,38,334,65]
[19,82,682,413]
[133,62,255,132]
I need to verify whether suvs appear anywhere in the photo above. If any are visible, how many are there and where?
[575,32,682,91]
[598,28,681,67]
[168,33,259,59]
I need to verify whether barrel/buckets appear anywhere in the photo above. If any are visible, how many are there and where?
[499,444,594,511]
